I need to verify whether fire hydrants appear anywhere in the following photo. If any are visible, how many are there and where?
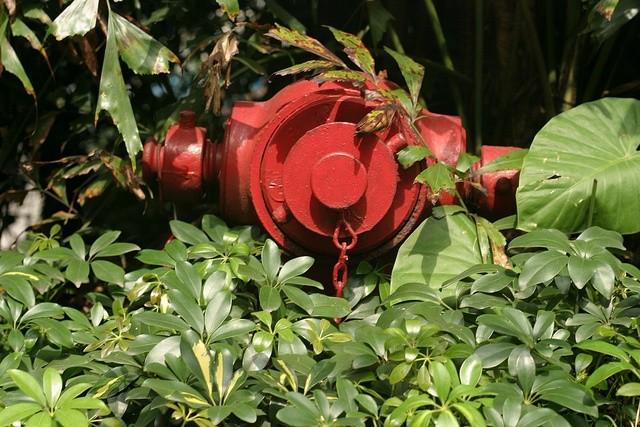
[142,71,531,322]
